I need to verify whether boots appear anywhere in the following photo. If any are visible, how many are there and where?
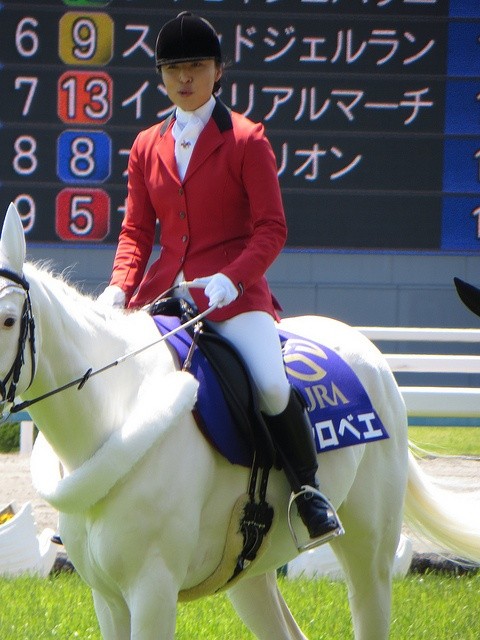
[257,384,339,539]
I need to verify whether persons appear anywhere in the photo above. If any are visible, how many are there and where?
[98,14,339,539]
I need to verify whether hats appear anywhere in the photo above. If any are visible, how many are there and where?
[156,11,220,67]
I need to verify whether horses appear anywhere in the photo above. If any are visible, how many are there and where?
[0,199,480,640]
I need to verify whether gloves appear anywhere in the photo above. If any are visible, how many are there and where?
[96,285,125,308]
[193,273,239,308]
